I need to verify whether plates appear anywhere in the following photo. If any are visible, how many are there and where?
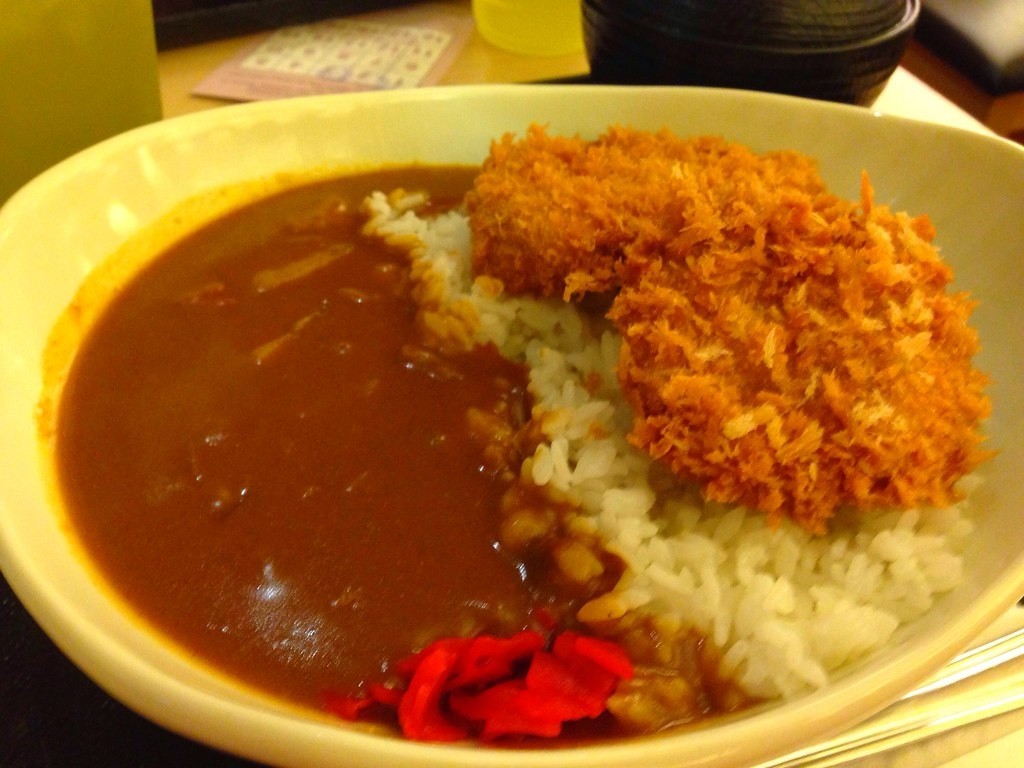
[0,83,1024,768]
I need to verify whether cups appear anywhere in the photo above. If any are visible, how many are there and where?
[0,0,164,205]
[471,0,583,58]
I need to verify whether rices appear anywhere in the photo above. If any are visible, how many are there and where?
[358,188,980,735]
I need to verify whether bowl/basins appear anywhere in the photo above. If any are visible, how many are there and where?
[580,0,922,107]
